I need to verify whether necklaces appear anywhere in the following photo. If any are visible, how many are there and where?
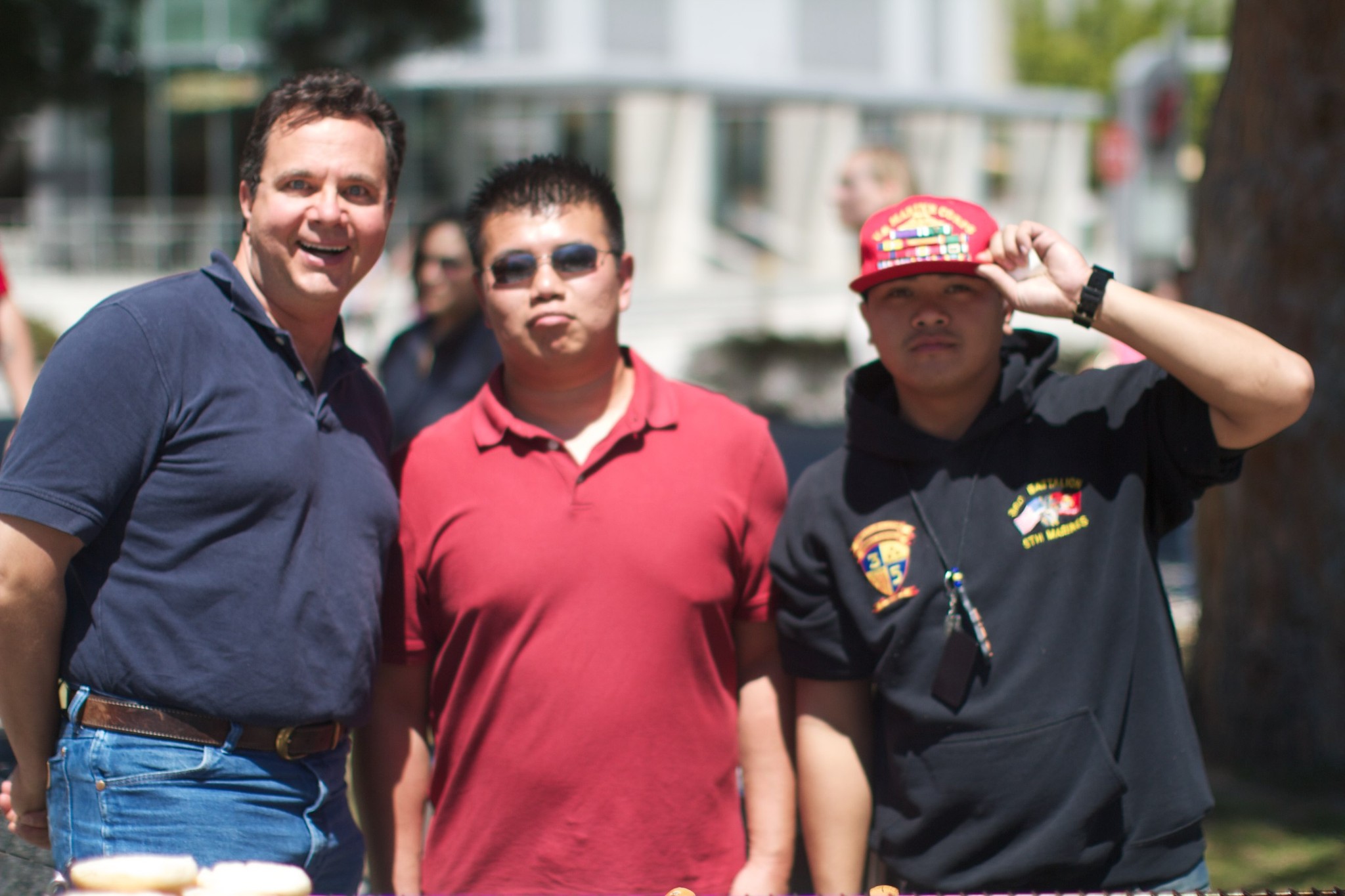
[890,435,994,712]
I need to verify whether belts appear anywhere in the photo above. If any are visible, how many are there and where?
[65,690,349,759]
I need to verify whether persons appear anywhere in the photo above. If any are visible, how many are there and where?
[353,156,796,896]
[0,70,406,896]
[768,194,1313,896]
[376,213,503,461]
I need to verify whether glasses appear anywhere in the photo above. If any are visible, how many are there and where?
[479,244,616,284]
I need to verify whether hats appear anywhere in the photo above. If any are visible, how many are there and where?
[849,195,999,293]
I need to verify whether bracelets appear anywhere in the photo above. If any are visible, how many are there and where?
[1072,265,1114,329]
